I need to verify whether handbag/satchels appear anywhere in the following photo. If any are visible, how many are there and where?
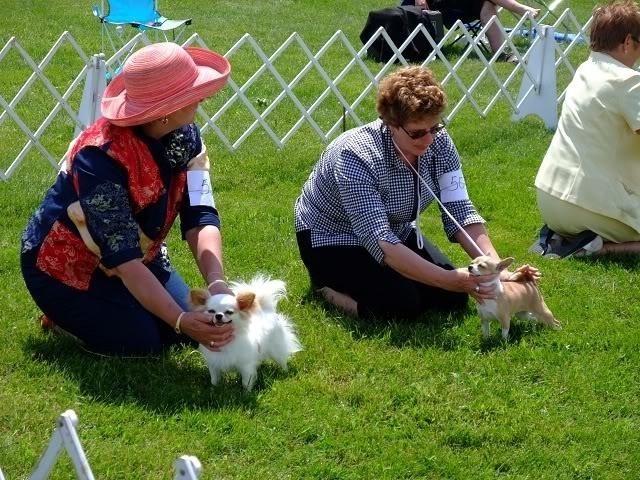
[360,5,443,63]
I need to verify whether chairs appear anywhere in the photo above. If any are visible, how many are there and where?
[93,0,192,66]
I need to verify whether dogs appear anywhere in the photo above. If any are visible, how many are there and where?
[468,252,562,341]
[188,271,305,393]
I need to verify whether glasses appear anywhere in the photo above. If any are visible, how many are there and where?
[399,117,446,139]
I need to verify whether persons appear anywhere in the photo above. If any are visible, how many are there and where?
[528,0,640,260]
[22,43,236,356]
[401,0,541,63]
[291,63,541,328]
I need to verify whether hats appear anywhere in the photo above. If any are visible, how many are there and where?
[100,42,231,127]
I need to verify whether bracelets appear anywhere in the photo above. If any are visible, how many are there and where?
[174,309,184,338]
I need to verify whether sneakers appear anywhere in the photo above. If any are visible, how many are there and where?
[40,315,55,329]
[528,223,564,256]
[544,230,603,260]
[496,49,520,62]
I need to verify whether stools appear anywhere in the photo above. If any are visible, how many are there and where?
[449,10,490,56]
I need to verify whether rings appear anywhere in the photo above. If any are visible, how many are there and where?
[208,341,215,348]
[476,285,480,292]
